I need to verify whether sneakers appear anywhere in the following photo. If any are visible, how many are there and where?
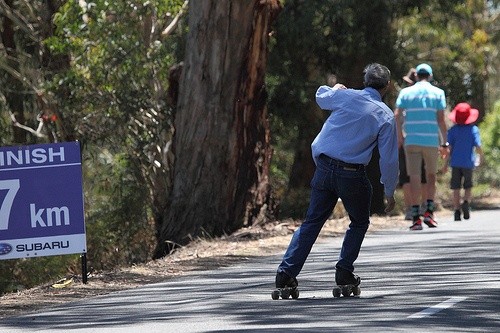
[410,220,422,230]
[423,212,437,227]
[453,208,461,220]
[461,202,470,219]
[405,208,413,220]
[419,207,427,216]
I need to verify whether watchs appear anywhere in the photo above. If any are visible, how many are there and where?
[440,142,449,147]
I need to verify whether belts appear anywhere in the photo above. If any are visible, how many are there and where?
[320,153,363,172]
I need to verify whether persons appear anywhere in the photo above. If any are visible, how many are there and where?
[272,63,399,300]
[441,102,484,221]
[394,63,450,230]
[394,68,427,220]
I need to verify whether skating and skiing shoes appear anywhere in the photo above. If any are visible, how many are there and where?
[333,267,362,297]
[271,273,299,300]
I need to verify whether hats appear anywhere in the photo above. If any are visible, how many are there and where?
[416,63,432,74]
[402,67,417,84]
[448,103,479,125]
